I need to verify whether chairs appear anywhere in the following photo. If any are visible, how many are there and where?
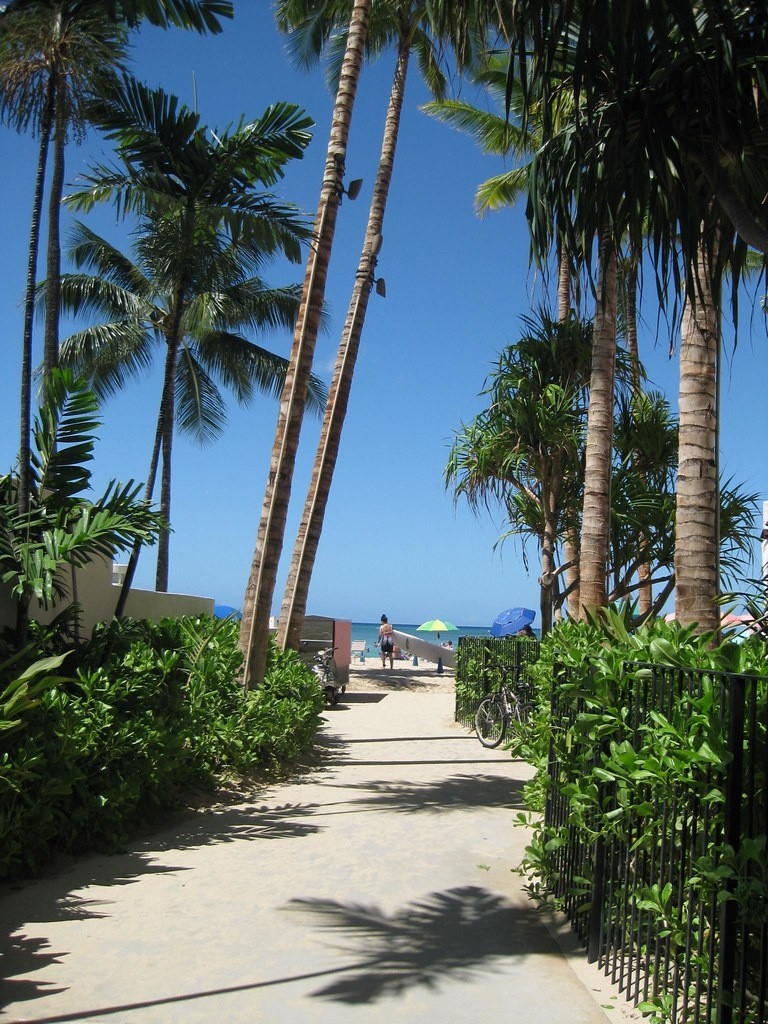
[350,640,367,662]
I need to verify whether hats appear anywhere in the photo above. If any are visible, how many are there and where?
[447,640,452,644]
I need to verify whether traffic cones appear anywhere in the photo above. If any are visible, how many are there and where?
[437,657,444,673]
[413,655,418,666]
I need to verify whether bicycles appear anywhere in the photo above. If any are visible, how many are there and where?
[473,664,546,749]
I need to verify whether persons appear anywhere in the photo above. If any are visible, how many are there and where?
[377,613,393,669]
[442,640,452,649]
[374,641,380,648]
[518,624,536,637]
[392,642,401,658]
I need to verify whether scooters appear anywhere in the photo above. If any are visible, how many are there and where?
[310,646,339,705]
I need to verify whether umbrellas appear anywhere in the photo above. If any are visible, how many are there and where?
[416,617,460,640]
[215,604,242,619]
[490,607,536,637]
